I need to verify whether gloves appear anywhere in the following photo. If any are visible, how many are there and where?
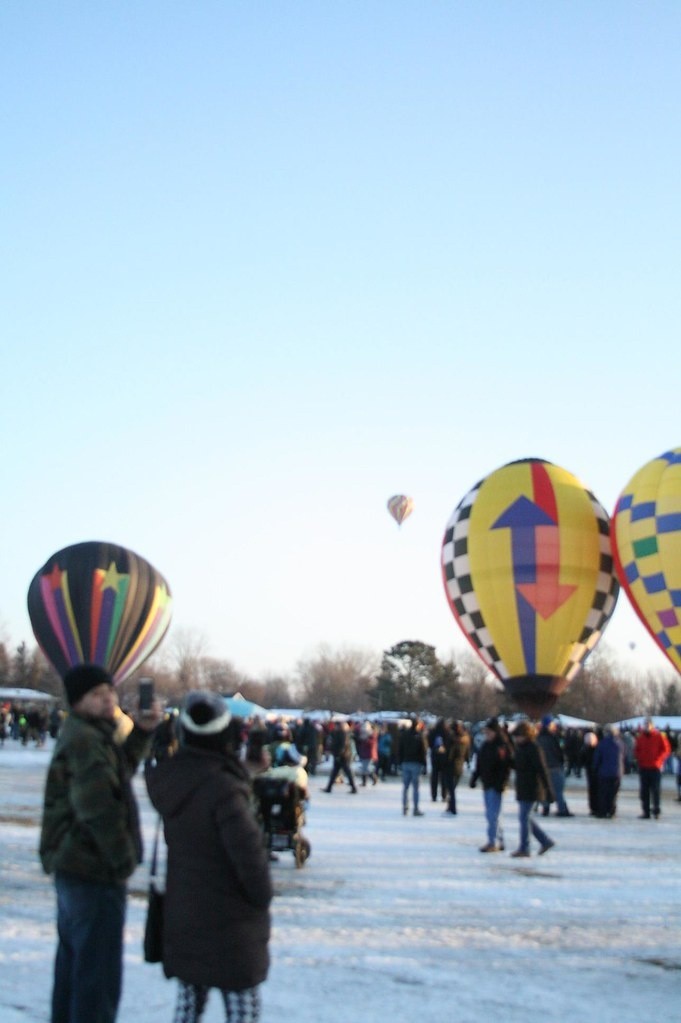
[470,778,477,788]
[546,792,556,803]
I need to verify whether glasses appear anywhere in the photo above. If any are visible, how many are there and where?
[514,728,525,736]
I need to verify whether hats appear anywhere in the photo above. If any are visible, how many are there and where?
[274,742,308,766]
[64,664,115,706]
[178,688,232,736]
[519,720,543,742]
[486,717,500,732]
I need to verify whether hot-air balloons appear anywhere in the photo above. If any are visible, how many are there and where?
[440,458,620,732]
[388,494,413,527]
[25,540,172,694]
[608,445,679,678]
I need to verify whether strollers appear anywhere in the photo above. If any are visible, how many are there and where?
[251,765,311,867]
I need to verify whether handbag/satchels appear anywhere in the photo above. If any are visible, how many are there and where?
[143,882,167,963]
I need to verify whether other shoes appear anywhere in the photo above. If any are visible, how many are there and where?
[538,842,554,855]
[480,844,504,852]
[512,851,530,857]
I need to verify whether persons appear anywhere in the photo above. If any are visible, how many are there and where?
[509,719,557,858]
[1,662,680,818]
[142,688,275,1023]
[37,664,162,1023]
[468,716,515,853]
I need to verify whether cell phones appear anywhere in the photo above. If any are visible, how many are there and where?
[137,674,157,721]
